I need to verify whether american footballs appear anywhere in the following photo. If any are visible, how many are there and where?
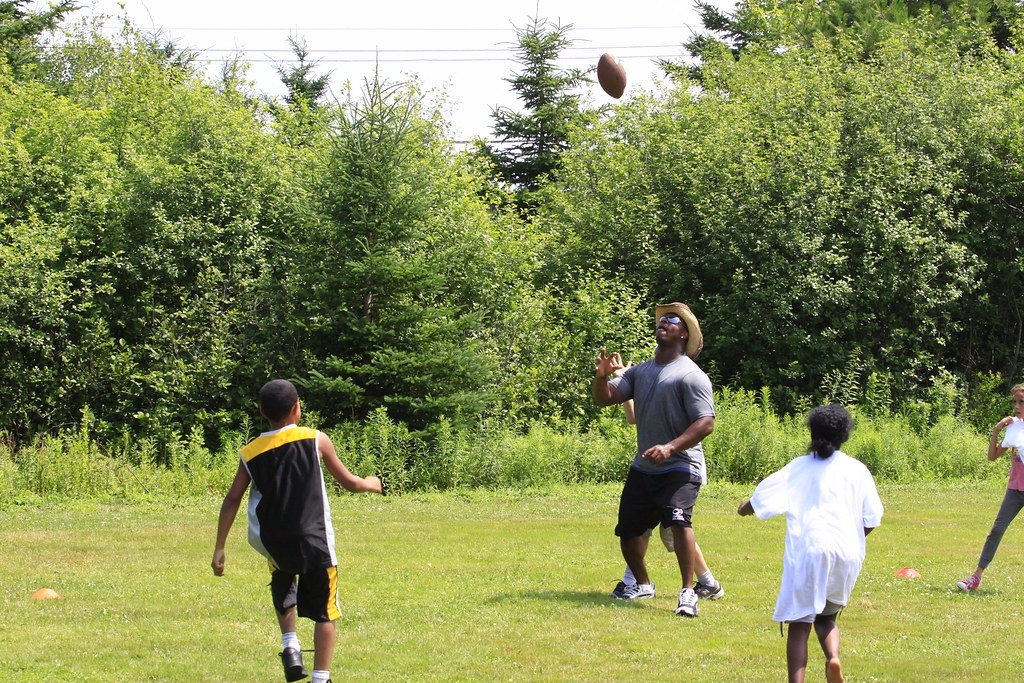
[597,52,627,99]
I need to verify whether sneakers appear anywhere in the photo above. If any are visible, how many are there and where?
[610,579,656,601]
[676,587,698,617]
[278,647,308,683]
[956,573,980,591]
[692,580,725,600]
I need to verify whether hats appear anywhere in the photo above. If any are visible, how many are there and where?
[654,302,703,361]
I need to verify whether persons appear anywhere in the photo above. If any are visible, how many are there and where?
[606,344,725,603]
[737,405,884,683]
[955,381,1024,592]
[211,379,382,681]
[585,304,716,618]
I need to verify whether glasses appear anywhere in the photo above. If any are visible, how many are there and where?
[659,316,689,333]
